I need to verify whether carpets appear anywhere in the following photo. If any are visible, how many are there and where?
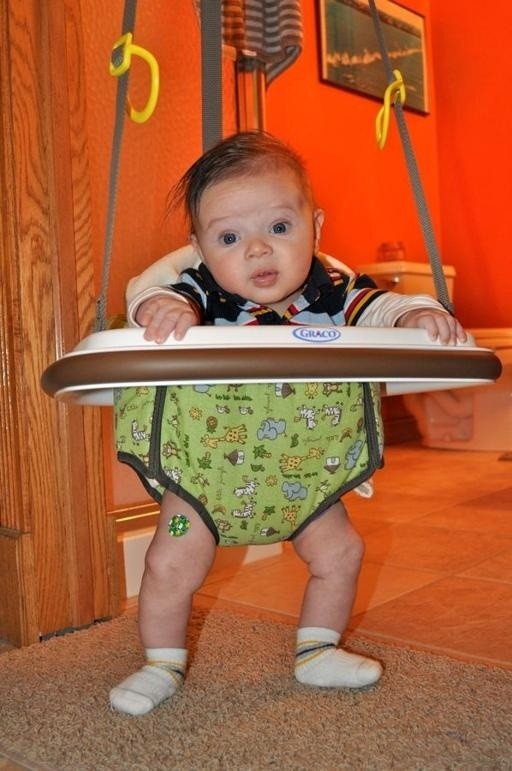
[0,606,512,771]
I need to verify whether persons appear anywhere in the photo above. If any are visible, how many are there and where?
[107,127,468,716]
